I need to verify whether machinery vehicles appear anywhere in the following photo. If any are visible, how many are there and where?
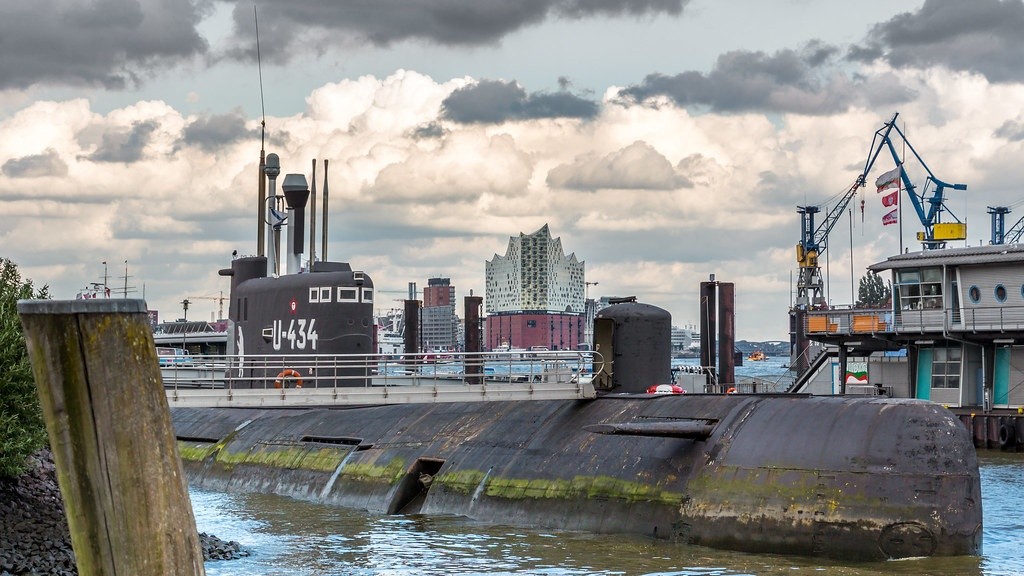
[795,171,866,312]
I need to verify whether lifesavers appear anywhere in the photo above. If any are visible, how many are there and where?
[273,369,303,388]
[646,383,683,395]
[998,424,1013,447]
[727,387,737,394]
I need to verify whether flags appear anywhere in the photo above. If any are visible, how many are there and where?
[882,210,897,225]
[875,167,900,193]
[882,191,898,207]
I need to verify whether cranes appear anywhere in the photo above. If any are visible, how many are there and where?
[188,291,230,319]
